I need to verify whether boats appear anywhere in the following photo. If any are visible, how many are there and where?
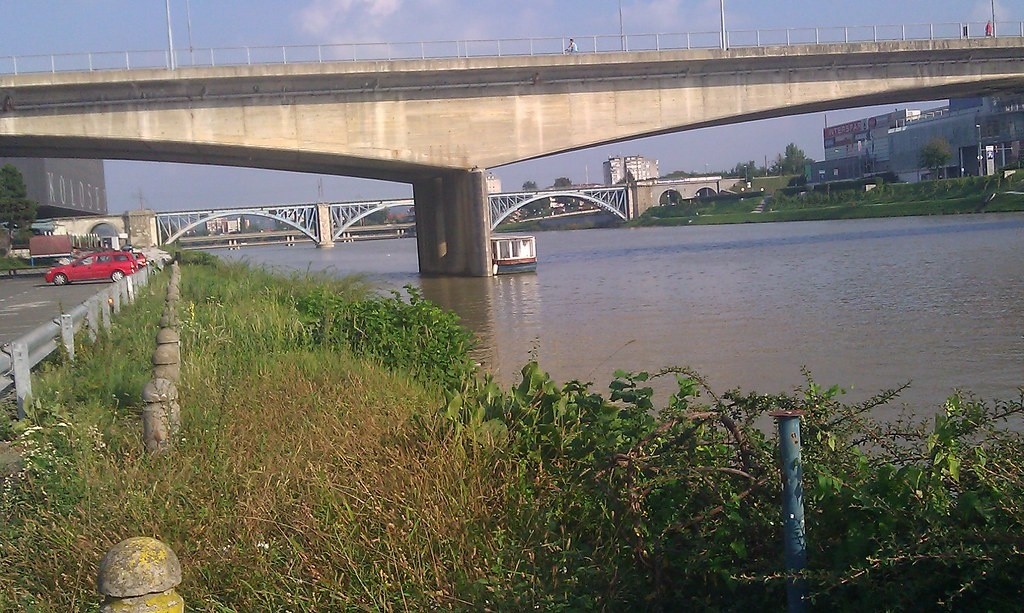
[491,235,537,275]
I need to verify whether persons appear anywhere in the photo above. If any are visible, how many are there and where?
[565,38,578,52]
[985,19,993,35]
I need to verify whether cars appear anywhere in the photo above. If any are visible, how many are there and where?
[132,252,147,270]
[46,251,139,284]
